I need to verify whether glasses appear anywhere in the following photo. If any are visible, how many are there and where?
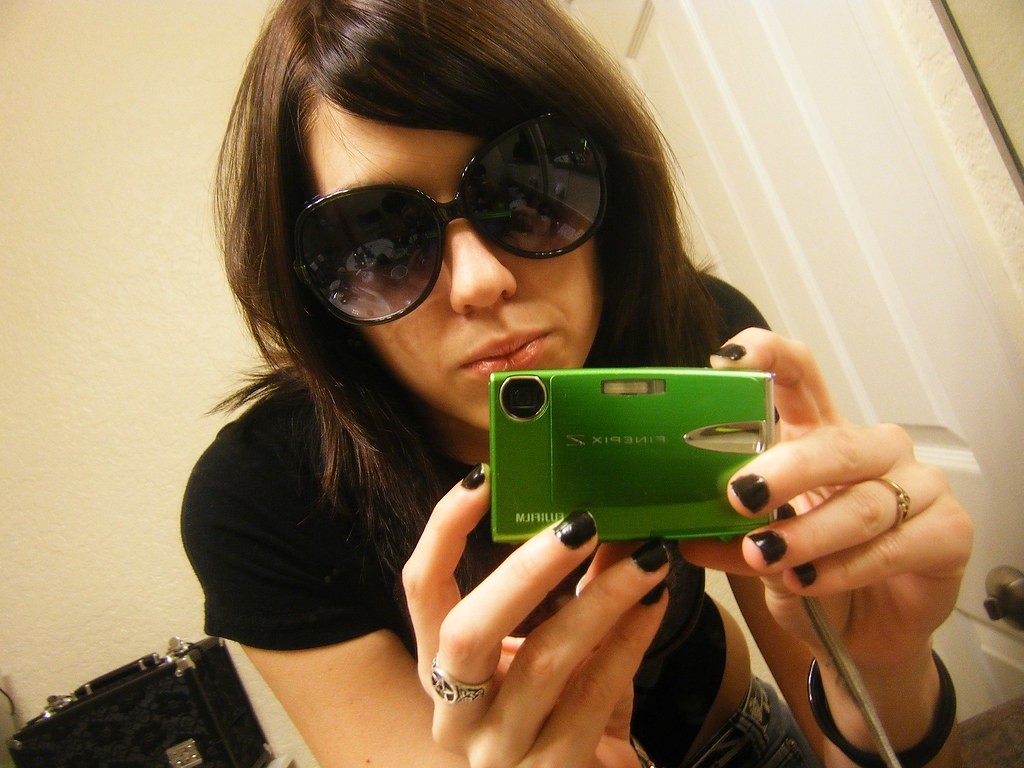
[293,110,607,327]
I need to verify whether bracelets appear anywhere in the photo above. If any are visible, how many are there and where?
[806,648,955,768]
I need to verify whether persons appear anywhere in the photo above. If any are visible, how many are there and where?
[175,0,980,768]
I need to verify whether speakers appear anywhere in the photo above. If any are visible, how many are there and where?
[7,637,273,768]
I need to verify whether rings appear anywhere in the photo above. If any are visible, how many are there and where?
[872,476,911,529]
[430,651,498,705]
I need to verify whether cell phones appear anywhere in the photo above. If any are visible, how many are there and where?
[489,366,776,542]
